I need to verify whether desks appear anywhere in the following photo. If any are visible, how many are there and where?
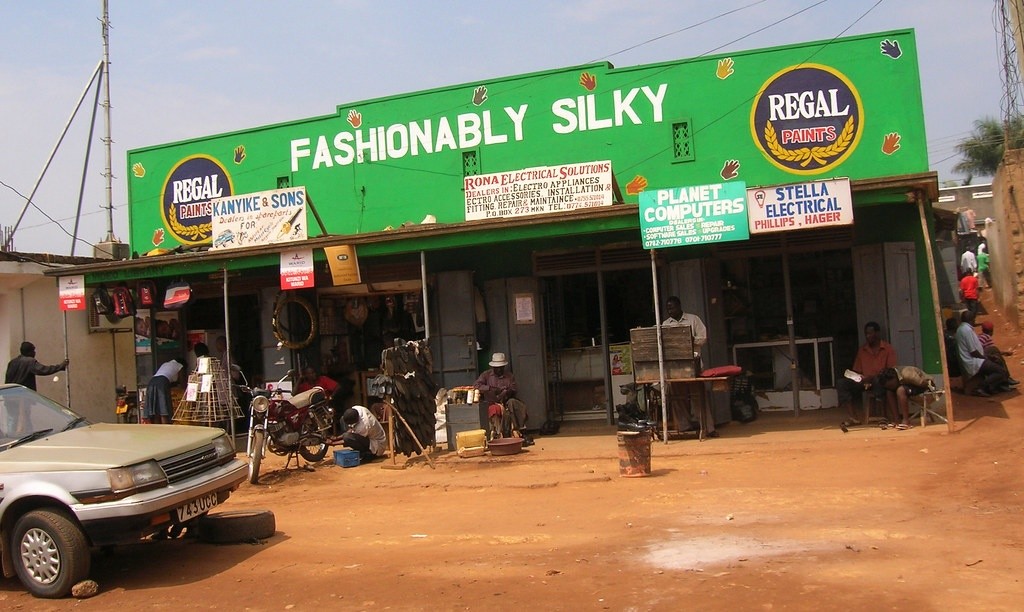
[733,336,838,411]
[635,377,727,442]
[360,370,386,408]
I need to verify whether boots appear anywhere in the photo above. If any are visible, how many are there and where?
[616,401,657,432]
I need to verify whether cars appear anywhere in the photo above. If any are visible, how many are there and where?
[0,381,250,596]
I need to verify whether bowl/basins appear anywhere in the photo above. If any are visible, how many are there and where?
[487,437,525,455]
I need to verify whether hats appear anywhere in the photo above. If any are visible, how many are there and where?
[341,409,359,425]
[489,353,508,367]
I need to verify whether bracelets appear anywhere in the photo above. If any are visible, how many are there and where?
[328,395,334,401]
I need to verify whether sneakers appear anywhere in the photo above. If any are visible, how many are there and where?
[1008,377,1019,385]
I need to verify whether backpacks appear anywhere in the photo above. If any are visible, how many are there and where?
[91,283,114,315]
[136,279,159,309]
[111,282,137,318]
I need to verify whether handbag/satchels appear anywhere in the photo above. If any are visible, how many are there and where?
[161,277,193,310]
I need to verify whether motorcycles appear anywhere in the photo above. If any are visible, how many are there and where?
[229,362,334,484]
[114,386,137,424]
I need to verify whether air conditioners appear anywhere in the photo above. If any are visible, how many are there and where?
[88,293,135,331]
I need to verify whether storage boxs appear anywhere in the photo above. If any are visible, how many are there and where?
[333,449,360,467]
[629,325,700,380]
[444,402,486,458]
[730,369,771,394]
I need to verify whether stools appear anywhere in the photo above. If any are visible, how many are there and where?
[909,389,957,427]
[864,390,890,424]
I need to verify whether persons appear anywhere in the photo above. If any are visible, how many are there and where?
[662,296,720,437]
[3,342,70,438]
[877,366,936,429]
[944,311,1020,397]
[839,321,896,427]
[142,358,188,424]
[954,236,993,325]
[194,337,243,433]
[757,317,812,390]
[474,353,535,445]
[371,333,397,369]
[292,367,341,438]
[612,355,624,375]
[326,405,387,460]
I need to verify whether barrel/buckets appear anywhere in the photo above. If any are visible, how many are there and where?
[616,430,652,477]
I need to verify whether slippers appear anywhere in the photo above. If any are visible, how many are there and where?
[840,419,861,427]
[885,423,914,430]
[878,417,888,427]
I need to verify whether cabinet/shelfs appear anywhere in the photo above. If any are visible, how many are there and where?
[706,249,858,368]
